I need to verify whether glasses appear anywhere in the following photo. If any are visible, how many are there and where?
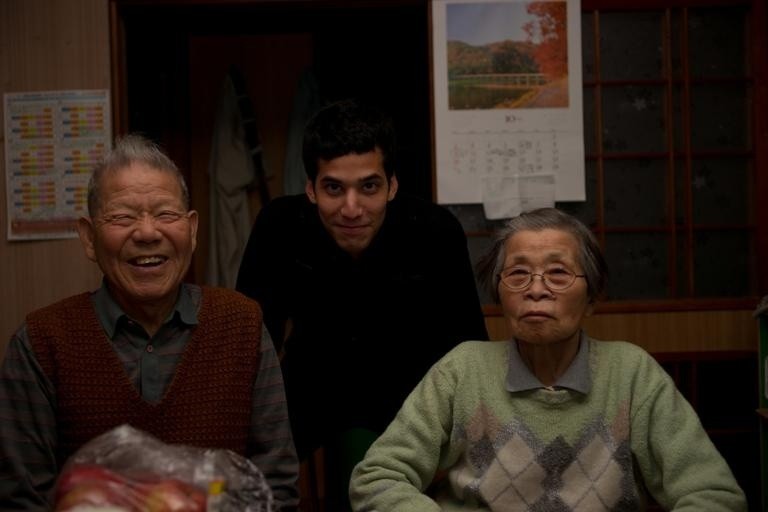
[495,264,586,291]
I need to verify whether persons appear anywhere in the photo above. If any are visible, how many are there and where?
[1,133,302,512]
[348,210,747,512]
[234,100,489,512]
